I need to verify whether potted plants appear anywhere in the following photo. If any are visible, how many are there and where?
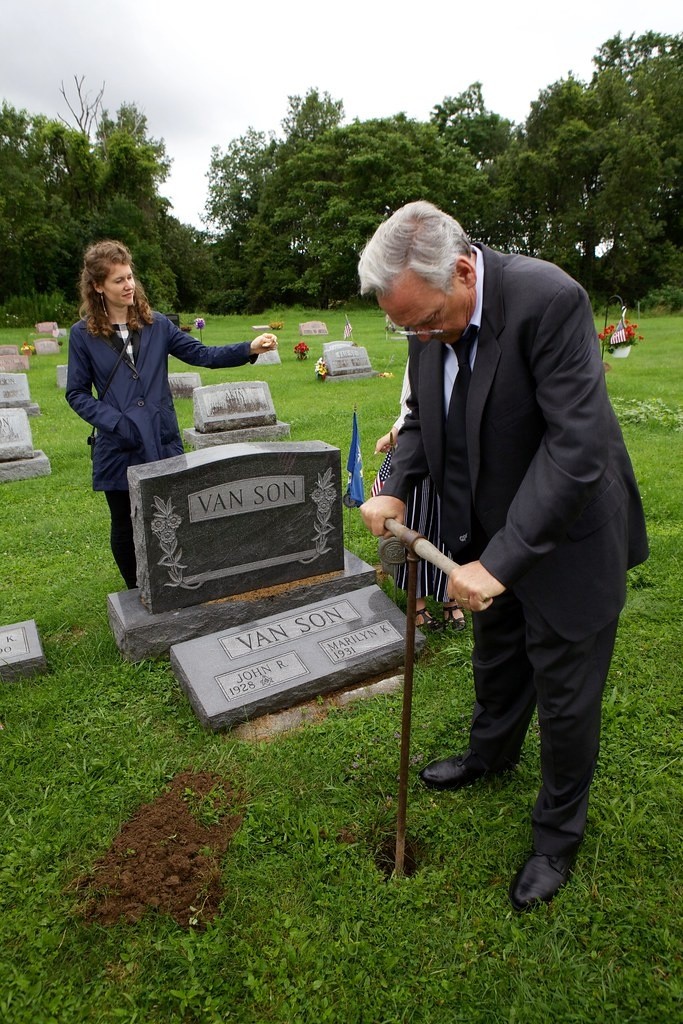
[609,344,633,358]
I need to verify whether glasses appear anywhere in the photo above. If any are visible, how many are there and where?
[384,268,453,337]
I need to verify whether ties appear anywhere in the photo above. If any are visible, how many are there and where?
[439,324,478,556]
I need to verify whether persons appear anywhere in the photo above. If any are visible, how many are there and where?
[374,357,466,632]
[358,200,654,913]
[65,240,280,592]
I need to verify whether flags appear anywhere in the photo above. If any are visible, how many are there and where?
[347,411,364,509]
[370,449,393,497]
[343,322,353,341]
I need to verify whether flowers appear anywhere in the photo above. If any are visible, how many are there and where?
[597,317,645,353]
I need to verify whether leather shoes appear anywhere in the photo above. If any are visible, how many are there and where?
[421,750,491,790]
[509,851,572,913]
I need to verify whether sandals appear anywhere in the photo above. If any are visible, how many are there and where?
[416,607,442,632]
[442,605,465,631]
